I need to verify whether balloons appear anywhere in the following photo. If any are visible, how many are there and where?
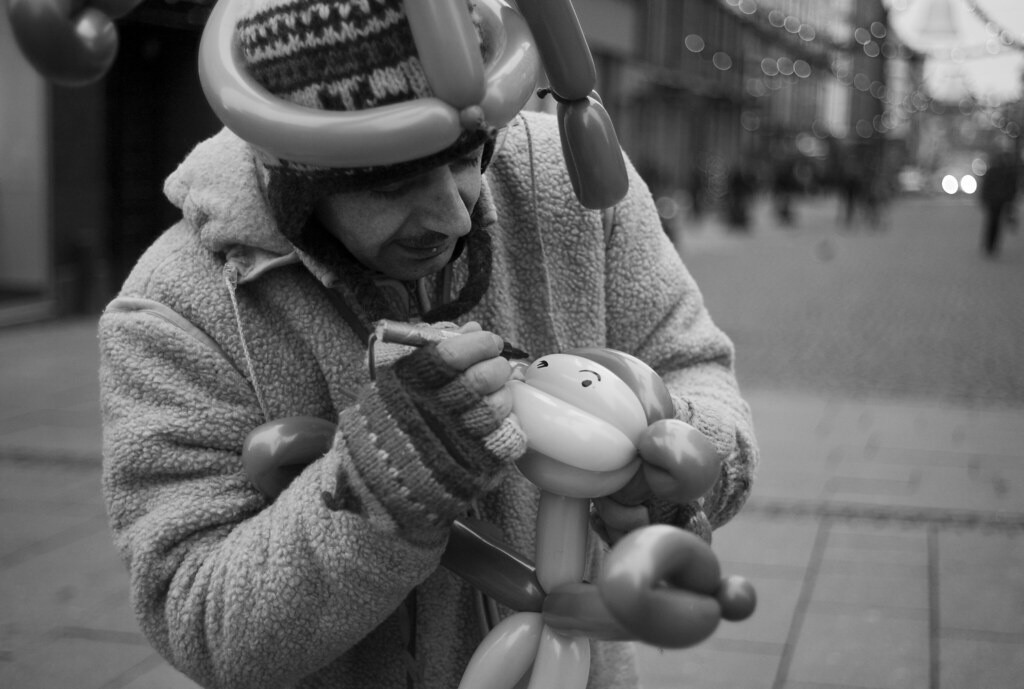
[199,0,628,210]
[243,347,758,689]
[7,0,138,87]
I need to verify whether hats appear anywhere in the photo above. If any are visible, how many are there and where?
[237,1,486,177]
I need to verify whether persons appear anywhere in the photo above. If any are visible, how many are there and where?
[97,0,759,689]
[972,150,1018,256]
[831,155,895,229]
[683,149,802,232]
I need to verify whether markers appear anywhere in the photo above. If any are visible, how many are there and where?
[376,319,529,361]
